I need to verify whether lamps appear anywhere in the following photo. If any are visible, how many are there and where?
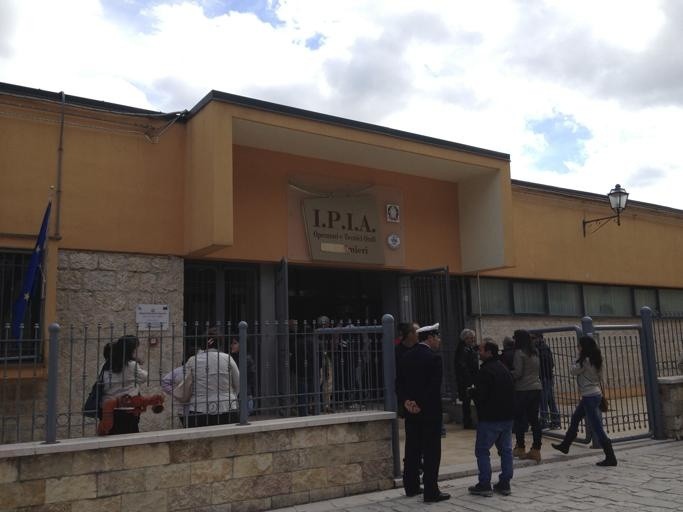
[583,183,629,237]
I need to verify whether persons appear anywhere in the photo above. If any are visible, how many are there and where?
[508,329,543,461]
[289,316,370,412]
[100,335,149,434]
[98,342,112,374]
[184,327,239,428]
[160,346,196,427]
[464,339,515,497]
[230,332,257,418]
[454,328,562,433]
[550,335,617,466]
[395,323,451,503]
[393,322,447,474]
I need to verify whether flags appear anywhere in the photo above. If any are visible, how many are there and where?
[9,199,51,341]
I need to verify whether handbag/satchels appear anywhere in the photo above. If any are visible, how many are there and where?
[173,368,192,402]
[600,397,608,412]
[85,382,102,417]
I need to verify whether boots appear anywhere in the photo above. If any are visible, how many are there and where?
[596,438,617,466]
[520,446,541,460]
[551,432,576,454]
[513,447,525,457]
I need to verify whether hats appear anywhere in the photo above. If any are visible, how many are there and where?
[415,322,440,335]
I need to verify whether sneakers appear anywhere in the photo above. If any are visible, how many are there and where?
[467,483,493,497]
[493,480,510,496]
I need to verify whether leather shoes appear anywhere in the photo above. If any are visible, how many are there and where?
[406,488,424,497]
[424,492,450,502]
